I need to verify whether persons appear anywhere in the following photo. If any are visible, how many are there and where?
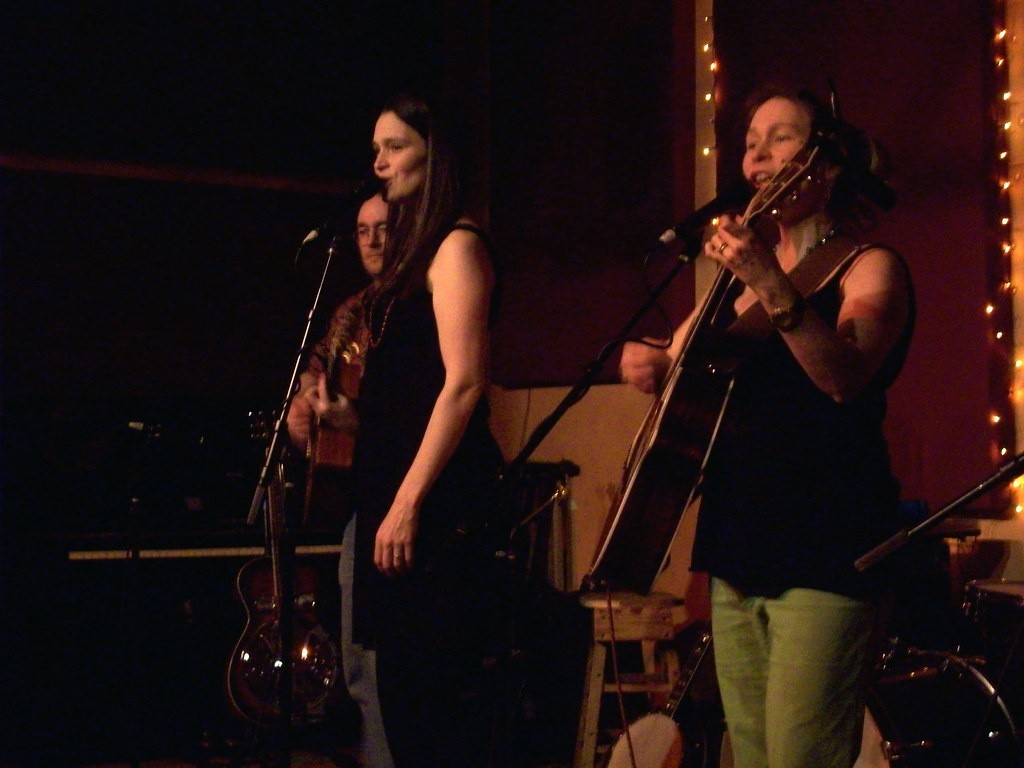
[617,79,917,768]
[285,87,517,768]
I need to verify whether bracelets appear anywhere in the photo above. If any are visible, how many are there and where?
[774,294,805,330]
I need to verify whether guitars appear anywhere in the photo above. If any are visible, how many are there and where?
[600,618,713,768]
[300,339,363,529]
[579,88,844,597]
[226,406,339,730]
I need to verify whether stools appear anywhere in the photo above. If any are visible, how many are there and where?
[574,589,684,768]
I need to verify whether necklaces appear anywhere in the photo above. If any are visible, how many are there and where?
[368,294,395,348]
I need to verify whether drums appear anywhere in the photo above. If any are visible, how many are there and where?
[960,578,1024,665]
[852,646,1024,768]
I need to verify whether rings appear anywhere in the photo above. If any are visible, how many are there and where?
[719,242,728,255]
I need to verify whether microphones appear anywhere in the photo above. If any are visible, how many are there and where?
[302,174,385,243]
[655,176,757,249]
